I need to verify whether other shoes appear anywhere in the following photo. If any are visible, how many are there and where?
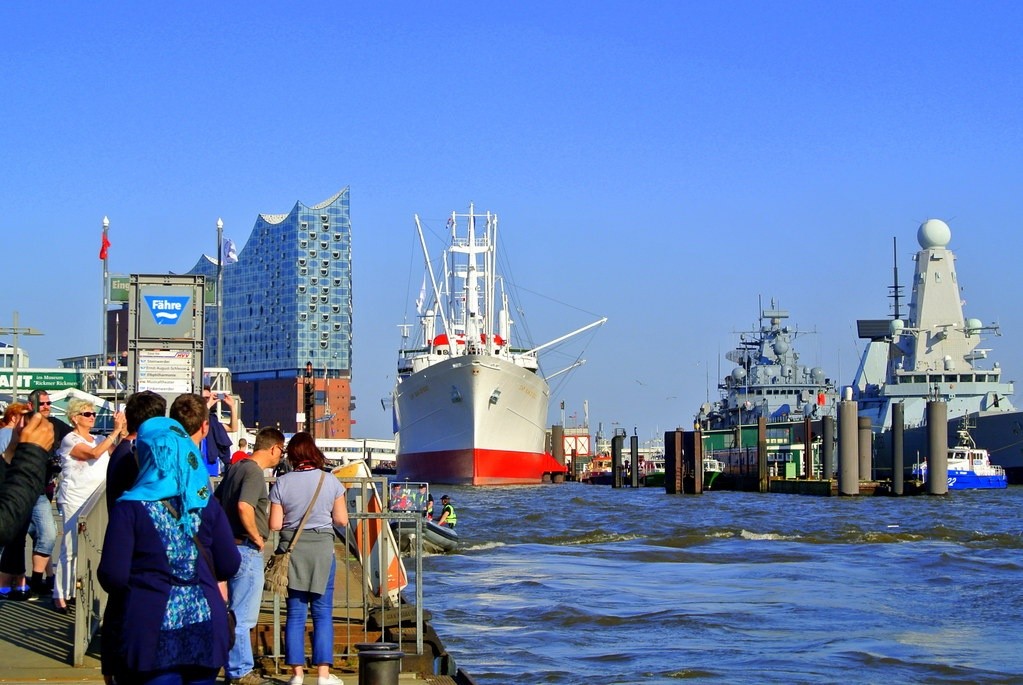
[0,591,28,600]
[53,599,67,614]
[230,672,270,685]
[288,677,303,685]
[31,583,51,597]
[318,676,344,685]
[47,574,54,588]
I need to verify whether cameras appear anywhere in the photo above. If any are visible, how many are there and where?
[217,394,226,399]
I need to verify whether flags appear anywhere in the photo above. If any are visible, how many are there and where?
[100,232,110,259]
[222,235,238,263]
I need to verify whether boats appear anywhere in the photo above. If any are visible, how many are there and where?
[703,455,727,491]
[381,200,609,488]
[96,365,258,458]
[912,410,1007,491]
[641,436,665,486]
[582,438,627,486]
[387,481,460,553]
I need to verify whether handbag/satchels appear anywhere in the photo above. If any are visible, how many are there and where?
[264,552,290,598]
[227,610,237,649]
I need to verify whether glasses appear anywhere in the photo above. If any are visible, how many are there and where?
[278,446,285,458]
[78,412,96,417]
[40,402,51,406]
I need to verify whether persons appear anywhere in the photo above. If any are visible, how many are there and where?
[1,349,457,684]
[96,415,242,685]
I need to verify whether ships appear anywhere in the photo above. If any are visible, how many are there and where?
[694,219,1023,483]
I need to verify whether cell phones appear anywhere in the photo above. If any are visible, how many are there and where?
[34,390,40,413]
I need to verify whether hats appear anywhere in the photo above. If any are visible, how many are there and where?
[440,495,449,500]
[418,484,425,488]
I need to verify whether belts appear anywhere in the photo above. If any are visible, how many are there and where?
[236,537,260,551]
